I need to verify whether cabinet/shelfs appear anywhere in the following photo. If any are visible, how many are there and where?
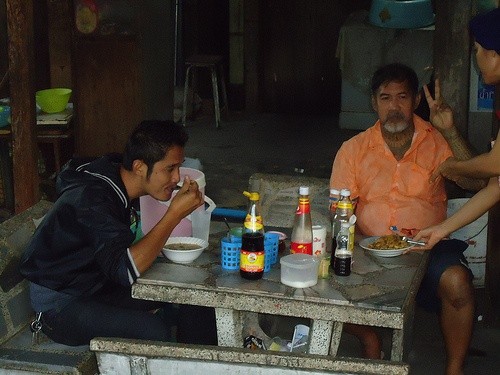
[75,33,140,159]
[332,10,437,132]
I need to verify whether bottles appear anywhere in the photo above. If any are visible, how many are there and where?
[290,185,313,256]
[331,190,357,276]
[240,190,265,280]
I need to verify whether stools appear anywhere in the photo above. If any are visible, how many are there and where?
[182,54,229,129]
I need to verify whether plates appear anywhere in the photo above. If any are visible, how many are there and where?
[360,237,415,257]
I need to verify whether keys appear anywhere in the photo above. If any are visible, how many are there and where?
[31,320,42,344]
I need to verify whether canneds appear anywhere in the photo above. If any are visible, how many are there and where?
[312,225,326,259]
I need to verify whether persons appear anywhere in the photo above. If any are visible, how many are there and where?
[330,62,488,375]
[20,118,205,343]
[403,6,500,255]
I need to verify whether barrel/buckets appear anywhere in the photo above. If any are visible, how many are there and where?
[447,198,490,286]
[141,166,217,243]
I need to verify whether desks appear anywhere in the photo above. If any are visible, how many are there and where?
[132,225,430,357]
[0,128,75,184]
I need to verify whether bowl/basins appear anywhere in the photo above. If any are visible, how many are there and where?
[280,253,319,288]
[0,106,11,128]
[161,237,209,264]
[368,0,436,29]
[35,88,73,114]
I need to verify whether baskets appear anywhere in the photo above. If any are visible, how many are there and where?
[220,229,279,272]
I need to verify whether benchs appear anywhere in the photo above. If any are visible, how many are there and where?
[248,173,333,232]
[90,336,409,375]
[0,199,99,375]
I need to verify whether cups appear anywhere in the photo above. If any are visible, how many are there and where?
[267,231,287,252]
[231,228,243,236]
[312,226,326,256]
